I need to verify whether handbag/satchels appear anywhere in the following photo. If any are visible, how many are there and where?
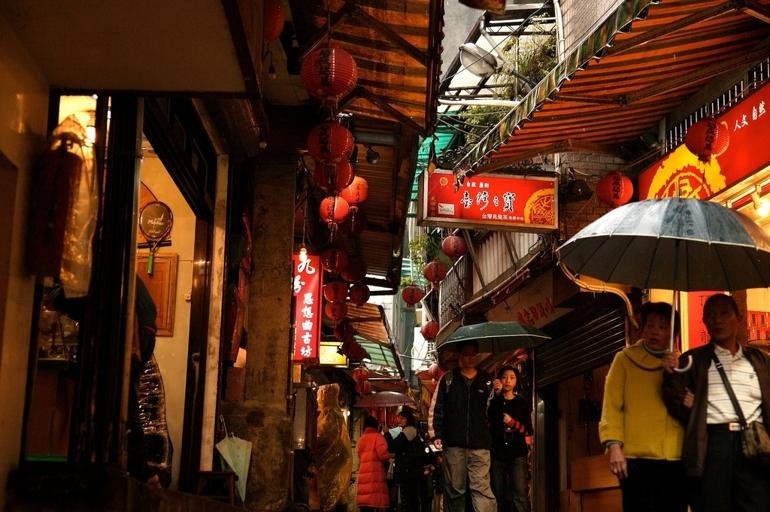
[494,440,514,465]
[741,421,770,458]
[387,471,398,493]
[420,441,437,466]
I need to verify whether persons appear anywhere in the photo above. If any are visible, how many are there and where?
[430,454,444,512]
[426,341,504,512]
[355,415,395,512]
[383,409,426,512]
[308,383,354,511]
[599,300,695,512]
[490,365,533,512]
[661,292,770,512]
[43,264,160,483]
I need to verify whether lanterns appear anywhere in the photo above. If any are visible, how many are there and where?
[301,43,370,322]
[420,320,439,340]
[402,286,423,305]
[442,233,468,261]
[428,363,444,389]
[684,117,730,164]
[353,365,399,426]
[595,171,634,207]
[423,259,447,284]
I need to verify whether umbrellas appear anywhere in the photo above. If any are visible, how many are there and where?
[552,194,770,373]
[215,414,253,510]
[435,319,552,377]
[353,390,417,425]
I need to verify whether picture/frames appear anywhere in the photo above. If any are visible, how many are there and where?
[136,250,180,338]
